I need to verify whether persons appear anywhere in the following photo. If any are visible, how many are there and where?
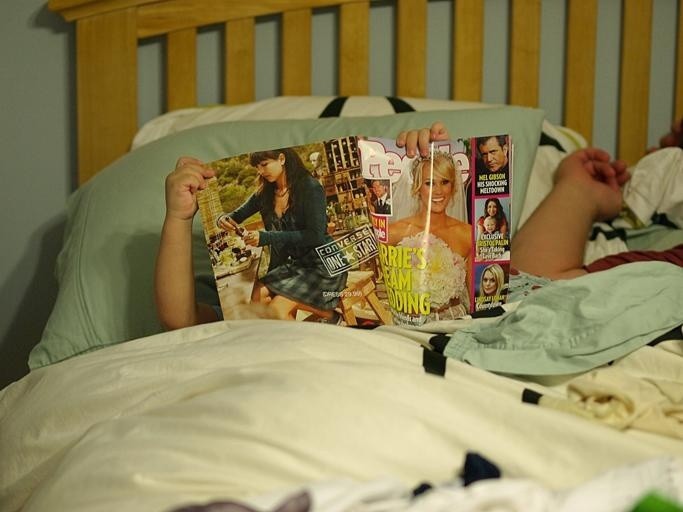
[509,114,682,281]
[151,121,449,331]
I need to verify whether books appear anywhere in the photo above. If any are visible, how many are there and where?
[194,130,516,332]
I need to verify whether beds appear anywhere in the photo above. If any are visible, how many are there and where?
[37,0,682,512]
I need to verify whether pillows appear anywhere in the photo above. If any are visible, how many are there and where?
[28,94,633,367]
